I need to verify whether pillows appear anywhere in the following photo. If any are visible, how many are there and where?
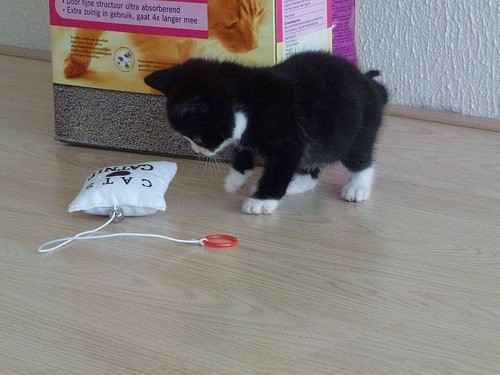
[66,161,178,218]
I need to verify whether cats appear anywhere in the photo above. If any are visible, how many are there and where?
[63,1,267,85]
[143,50,389,215]
